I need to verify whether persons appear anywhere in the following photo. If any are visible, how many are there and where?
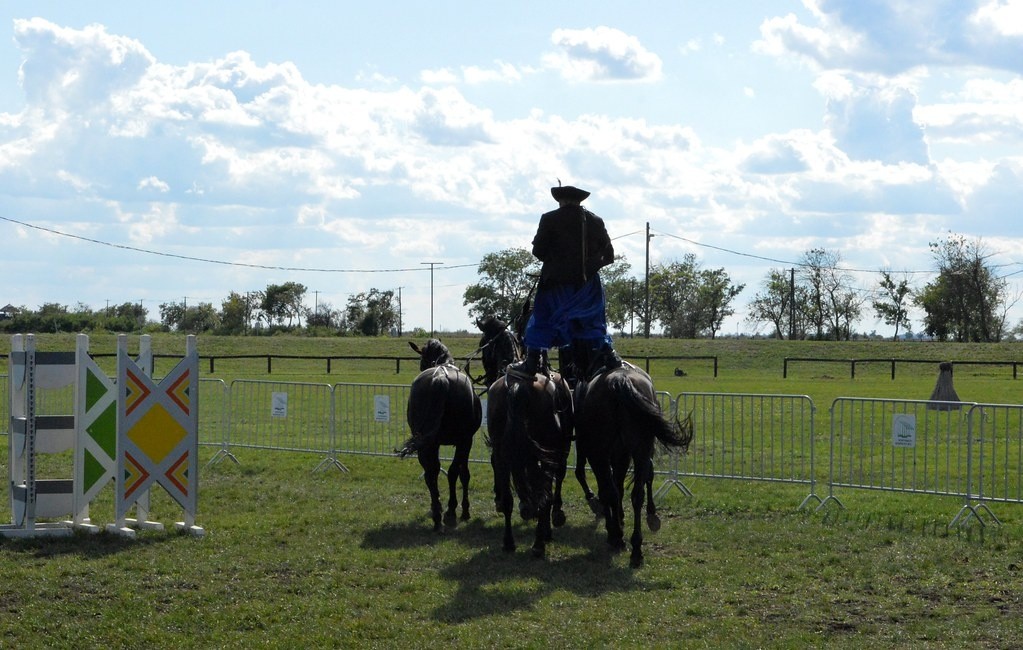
[504,178,624,380]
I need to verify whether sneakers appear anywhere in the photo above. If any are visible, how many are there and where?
[506,360,538,381]
[604,350,623,369]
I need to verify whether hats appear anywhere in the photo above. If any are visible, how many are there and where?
[550,186,590,202]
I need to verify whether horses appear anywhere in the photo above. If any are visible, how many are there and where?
[572,357,694,562]
[392,338,483,530]
[476,314,574,560]
